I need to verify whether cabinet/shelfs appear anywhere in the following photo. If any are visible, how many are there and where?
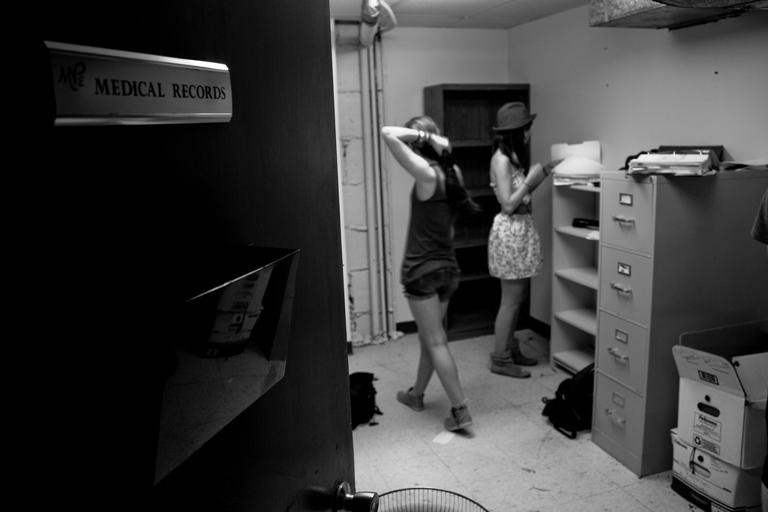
[423,82,531,341]
[592,166,767,479]
[548,172,601,374]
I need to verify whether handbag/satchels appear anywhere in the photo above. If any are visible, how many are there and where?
[541,361,595,439]
[349,372,381,429]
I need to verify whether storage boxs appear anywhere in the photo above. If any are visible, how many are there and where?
[669,316,767,512]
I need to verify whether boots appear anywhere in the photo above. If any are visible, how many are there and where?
[510,338,538,366]
[490,349,530,378]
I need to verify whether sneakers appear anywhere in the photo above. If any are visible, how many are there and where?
[397,387,424,412]
[445,405,472,430]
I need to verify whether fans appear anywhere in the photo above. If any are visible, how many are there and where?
[375,487,490,512]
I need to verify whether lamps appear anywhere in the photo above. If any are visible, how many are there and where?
[358,0,381,46]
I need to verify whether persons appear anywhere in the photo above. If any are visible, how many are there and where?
[484,101,553,380]
[377,113,482,434]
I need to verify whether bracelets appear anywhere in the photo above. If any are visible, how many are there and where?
[416,131,432,145]
[522,180,533,190]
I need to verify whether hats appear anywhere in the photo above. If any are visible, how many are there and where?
[492,102,536,132]
